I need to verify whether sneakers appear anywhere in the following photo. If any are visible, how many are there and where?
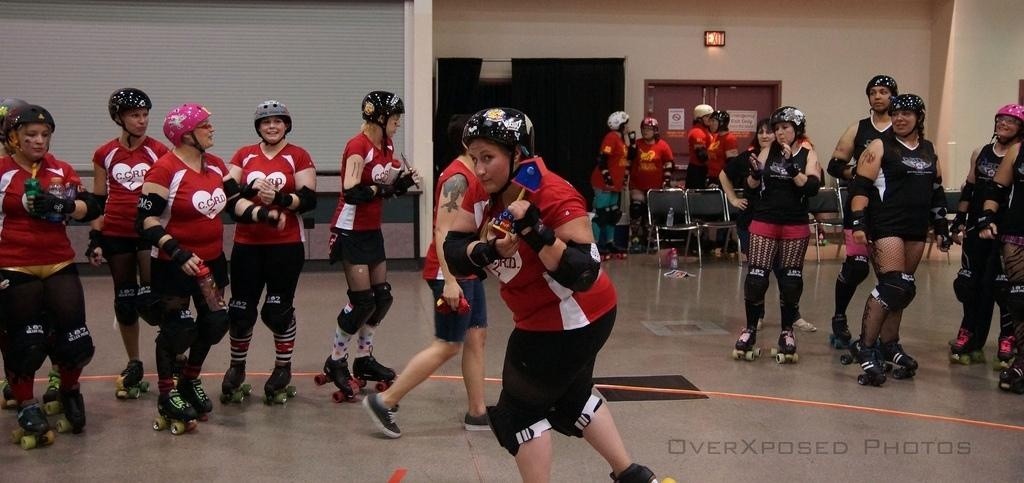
[792,319,817,332]
[464,412,491,431]
[363,394,402,438]
[757,319,764,331]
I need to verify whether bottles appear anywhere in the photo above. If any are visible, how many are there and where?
[665,206,674,226]
[194,263,224,314]
[22,175,83,224]
[665,247,680,269]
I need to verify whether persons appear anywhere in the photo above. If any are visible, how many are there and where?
[135,102,288,432]
[0,104,102,447]
[589,73,1023,394]
[1,94,71,408]
[220,100,316,403]
[363,109,497,439]
[316,91,422,403]
[86,87,159,398]
[444,104,656,481]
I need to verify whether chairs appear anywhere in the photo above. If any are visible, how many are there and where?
[807,185,850,262]
[684,187,744,268]
[926,190,962,265]
[647,188,703,269]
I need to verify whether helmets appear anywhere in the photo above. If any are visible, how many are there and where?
[641,118,659,136]
[463,108,535,157]
[770,106,805,140]
[608,111,630,130]
[109,88,152,120]
[995,104,1024,122]
[163,104,211,147]
[254,100,289,121]
[693,104,730,132]
[867,75,897,99]
[0,97,55,144]
[362,91,405,118]
[888,94,925,117]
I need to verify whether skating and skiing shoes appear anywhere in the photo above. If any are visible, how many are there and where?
[314,355,362,401]
[3,367,86,450]
[610,463,676,483]
[829,315,918,384]
[733,328,761,361]
[116,355,297,435]
[949,323,1023,393]
[595,237,659,260]
[352,356,395,391]
[770,331,799,363]
